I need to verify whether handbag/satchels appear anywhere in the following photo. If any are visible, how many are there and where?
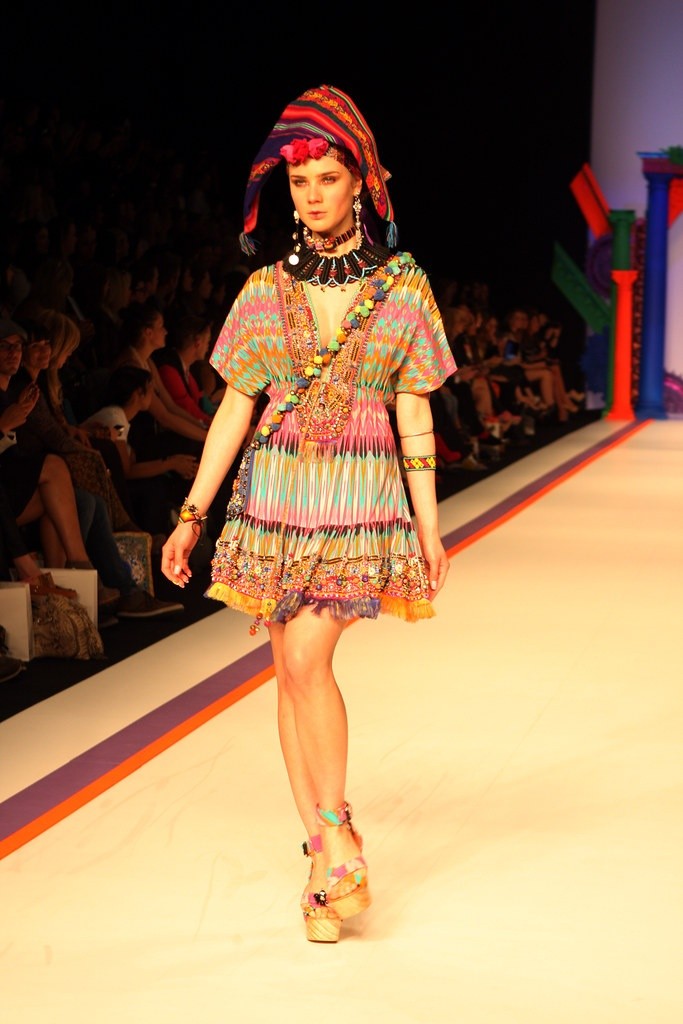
[33,585,111,660]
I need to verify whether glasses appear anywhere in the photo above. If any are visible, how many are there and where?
[28,341,50,348]
[0,340,25,353]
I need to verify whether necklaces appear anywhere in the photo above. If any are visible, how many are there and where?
[303,225,358,254]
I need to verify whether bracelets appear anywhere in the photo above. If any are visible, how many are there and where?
[398,430,434,438]
[176,499,208,537]
[402,455,436,471]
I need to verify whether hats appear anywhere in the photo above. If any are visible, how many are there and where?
[239,84,398,256]
[0,320,28,341]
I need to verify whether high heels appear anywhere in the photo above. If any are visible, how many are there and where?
[315,801,372,919]
[300,835,342,942]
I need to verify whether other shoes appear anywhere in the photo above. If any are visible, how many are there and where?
[568,389,584,403]
[524,426,535,435]
[32,585,77,599]
[117,589,184,617]
[478,435,501,446]
[446,459,488,474]
[0,656,21,683]
[448,444,474,464]
[99,616,118,629]
[65,566,119,606]
[22,662,28,671]
[151,534,166,555]
[564,403,579,413]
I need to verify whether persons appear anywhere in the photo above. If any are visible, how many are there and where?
[160,85,458,946]
[2,211,577,723]
